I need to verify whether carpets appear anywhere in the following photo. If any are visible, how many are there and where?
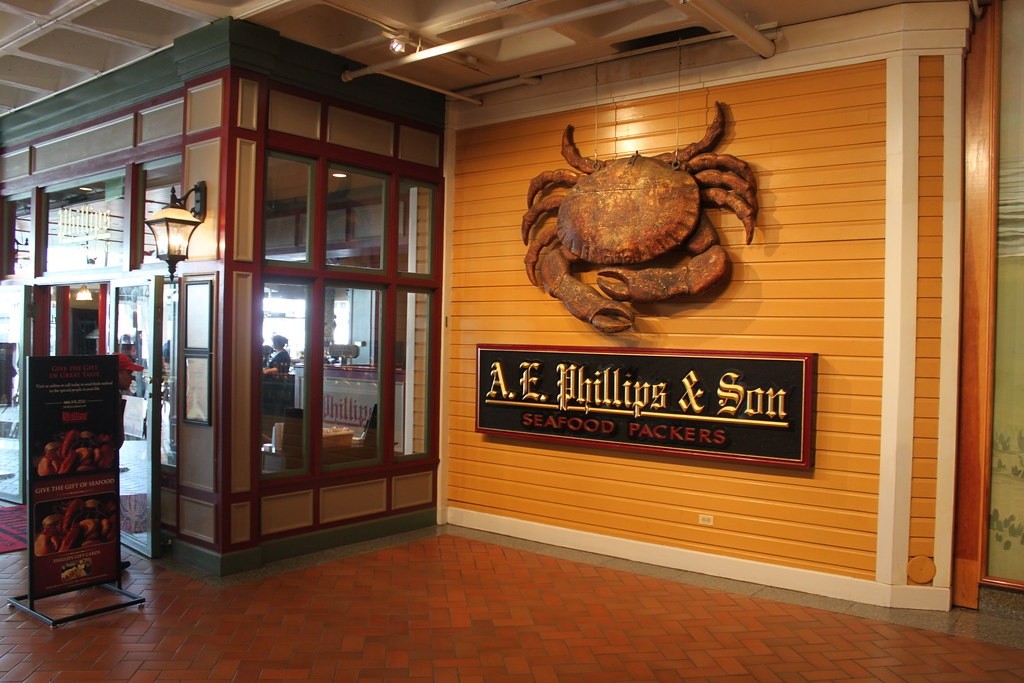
[0,504,27,555]
[121,495,148,534]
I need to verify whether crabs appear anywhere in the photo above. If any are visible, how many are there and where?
[521,101,759,334]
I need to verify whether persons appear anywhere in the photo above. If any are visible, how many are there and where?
[117,335,144,394]
[261,333,291,375]
[161,340,172,393]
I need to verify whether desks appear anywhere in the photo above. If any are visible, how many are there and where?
[261,437,365,474]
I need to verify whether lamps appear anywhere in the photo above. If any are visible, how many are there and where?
[75,285,92,301]
[58,204,111,243]
[143,180,207,283]
[389,30,409,54]
